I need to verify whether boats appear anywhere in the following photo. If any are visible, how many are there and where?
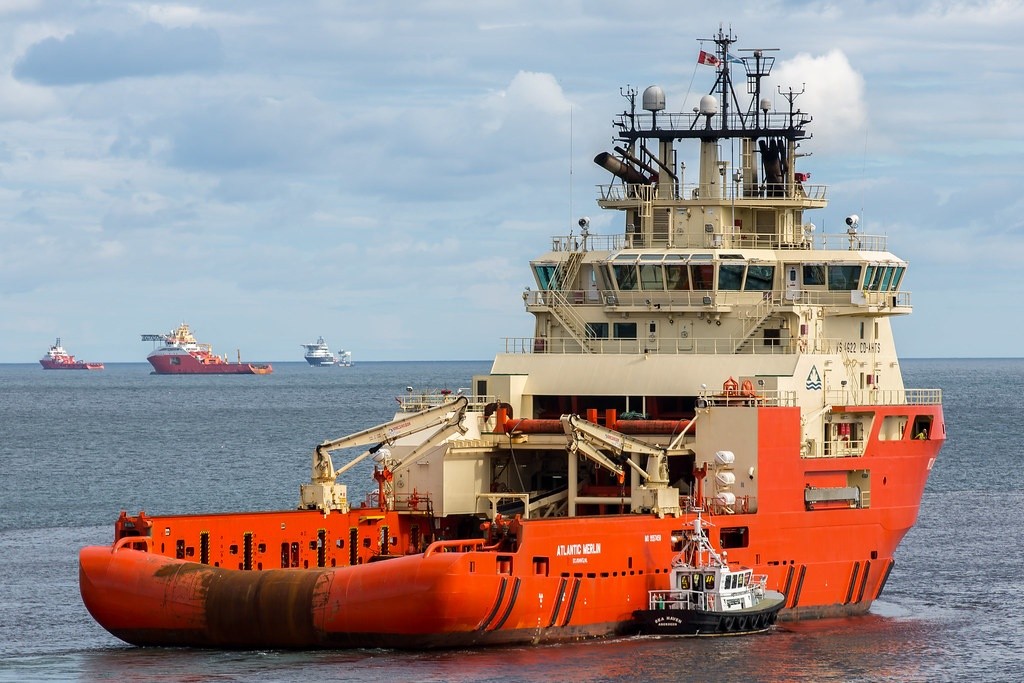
[632,483,788,634]
[39,338,104,369]
[300,336,354,367]
[77,21,952,652]
[147,320,272,374]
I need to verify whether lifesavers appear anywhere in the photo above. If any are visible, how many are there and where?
[708,594,716,609]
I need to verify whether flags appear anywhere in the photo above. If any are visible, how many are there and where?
[697,50,720,67]
[727,51,745,66]
